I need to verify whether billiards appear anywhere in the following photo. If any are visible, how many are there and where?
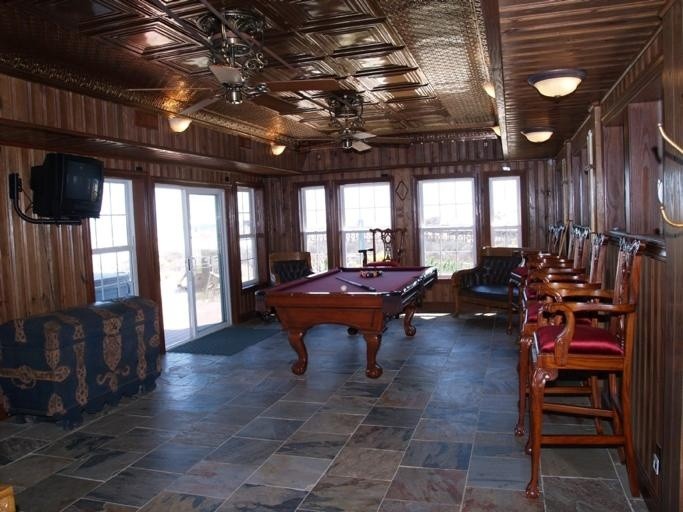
[361,270,384,277]
[341,285,348,291]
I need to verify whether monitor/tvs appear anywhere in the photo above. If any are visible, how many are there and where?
[29,151,105,221]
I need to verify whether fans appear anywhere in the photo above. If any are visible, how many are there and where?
[126,34,343,123]
[296,101,410,156]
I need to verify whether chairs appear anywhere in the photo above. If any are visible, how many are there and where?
[451,245,524,335]
[357,227,407,268]
[508,224,645,500]
[268,251,313,323]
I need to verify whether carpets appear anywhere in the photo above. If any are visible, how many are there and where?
[165,324,282,359]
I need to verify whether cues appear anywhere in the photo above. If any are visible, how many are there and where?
[336,276,376,291]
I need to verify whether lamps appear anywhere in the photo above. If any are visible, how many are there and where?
[527,68,587,99]
[520,126,555,144]
[482,81,496,98]
[272,145,287,156]
[492,126,501,136]
[168,116,191,131]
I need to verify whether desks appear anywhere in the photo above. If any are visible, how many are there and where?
[265,266,437,378]
